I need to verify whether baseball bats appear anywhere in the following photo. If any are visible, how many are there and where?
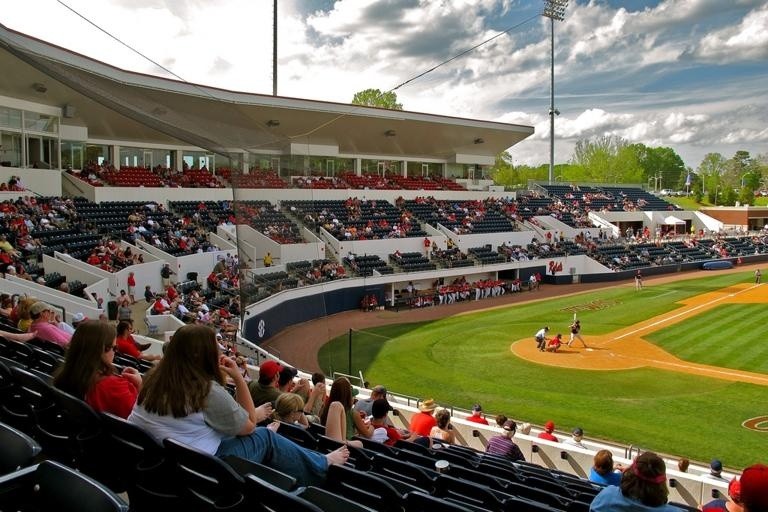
[573,313,576,326]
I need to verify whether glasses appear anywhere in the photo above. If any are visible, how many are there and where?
[107,343,120,352]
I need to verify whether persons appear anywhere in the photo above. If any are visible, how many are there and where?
[337,175,768,311]
[2,163,350,512]
[535,320,587,352]
[320,375,768,512]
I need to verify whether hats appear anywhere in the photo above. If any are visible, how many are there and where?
[28,302,53,314]
[572,427,584,436]
[740,462,768,503]
[711,459,723,470]
[257,358,298,383]
[544,419,555,431]
[728,478,740,501]
[415,397,440,413]
[471,403,482,412]
[502,420,517,431]
[72,311,88,325]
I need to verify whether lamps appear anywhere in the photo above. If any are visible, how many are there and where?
[385,130,395,137]
[266,119,279,127]
[474,137,483,144]
